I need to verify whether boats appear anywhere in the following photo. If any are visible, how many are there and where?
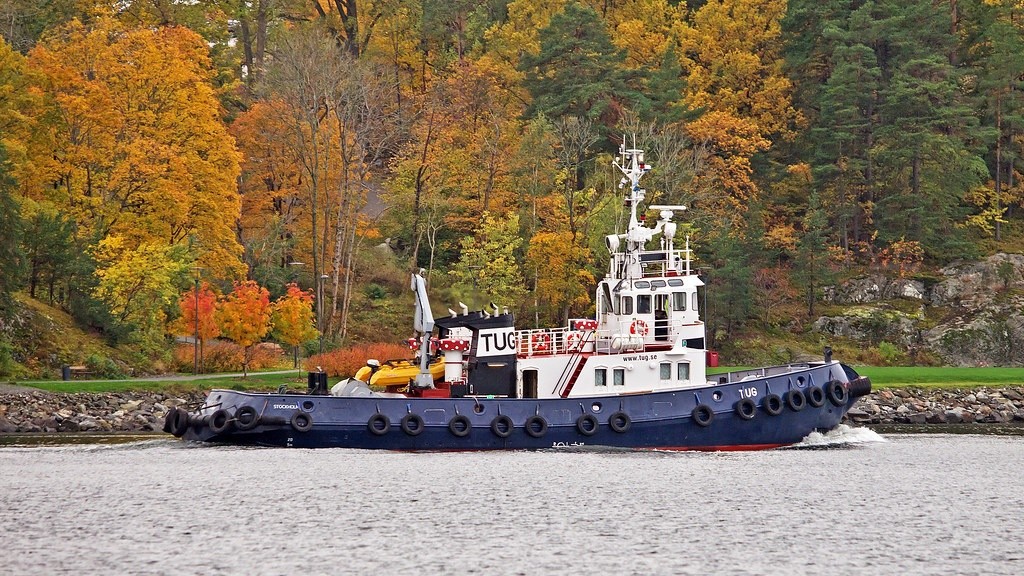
[353,351,445,386]
[165,128,873,450]
[384,380,452,398]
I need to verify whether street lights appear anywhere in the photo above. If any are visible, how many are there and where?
[467,266,484,311]
[288,262,305,365]
[699,267,713,347]
[190,269,204,375]
[807,226,821,316]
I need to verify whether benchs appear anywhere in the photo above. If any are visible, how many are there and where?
[70,365,94,379]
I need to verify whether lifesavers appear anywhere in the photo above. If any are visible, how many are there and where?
[567,333,584,350]
[208,408,233,436]
[161,405,190,438]
[401,413,425,437]
[368,412,391,436]
[827,379,849,408]
[490,415,514,439]
[532,332,550,351]
[233,405,259,432]
[690,404,714,427]
[786,389,807,413]
[290,411,314,434]
[525,415,548,438]
[807,385,826,408]
[630,320,649,336]
[609,411,632,434]
[448,414,474,438]
[762,394,785,416]
[576,413,600,437]
[736,398,758,420]
